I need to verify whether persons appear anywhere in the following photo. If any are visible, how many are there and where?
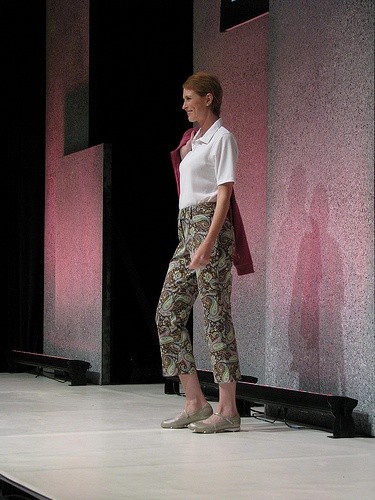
[155,71,241,436]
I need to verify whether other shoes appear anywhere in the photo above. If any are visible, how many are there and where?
[187,411,241,433]
[161,403,213,428]
